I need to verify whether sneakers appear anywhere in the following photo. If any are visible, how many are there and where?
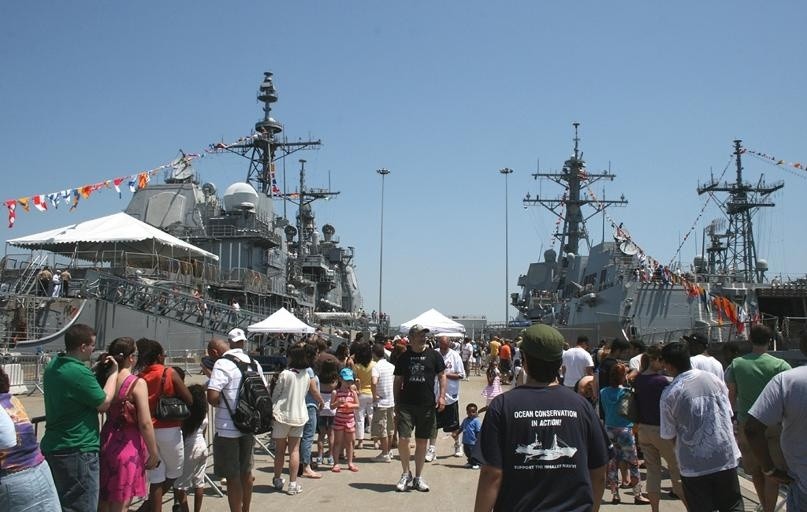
[302,470,322,478]
[331,464,341,472]
[349,463,360,472]
[396,470,413,491]
[327,458,334,465]
[454,440,464,456]
[355,440,393,462]
[285,483,303,495]
[272,476,286,489]
[425,448,436,462]
[413,476,429,492]
[612,497,620,504]
[633,495,651,504]
[316,457,323,465]
[464,461,480,469]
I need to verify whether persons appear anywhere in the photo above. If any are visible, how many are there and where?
[0,255,807,511]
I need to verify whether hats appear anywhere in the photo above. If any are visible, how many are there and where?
[520,323,565,362]
[339,367,355,383]
[409,324,430,337]
[683,332,708,346]
[227,328,248,343]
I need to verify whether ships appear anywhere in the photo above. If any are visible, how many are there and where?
[482,119,777,356]
[0,71,400,404]
[671,138,806,345]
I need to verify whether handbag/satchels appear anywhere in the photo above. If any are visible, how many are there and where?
[617,385,644,422]
[155,368,192,422]
[118,377,155,427]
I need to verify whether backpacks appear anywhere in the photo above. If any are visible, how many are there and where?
[217,354,274,435]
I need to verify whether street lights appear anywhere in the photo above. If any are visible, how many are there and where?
[500,167,513,339]
[375,167,389,336]
